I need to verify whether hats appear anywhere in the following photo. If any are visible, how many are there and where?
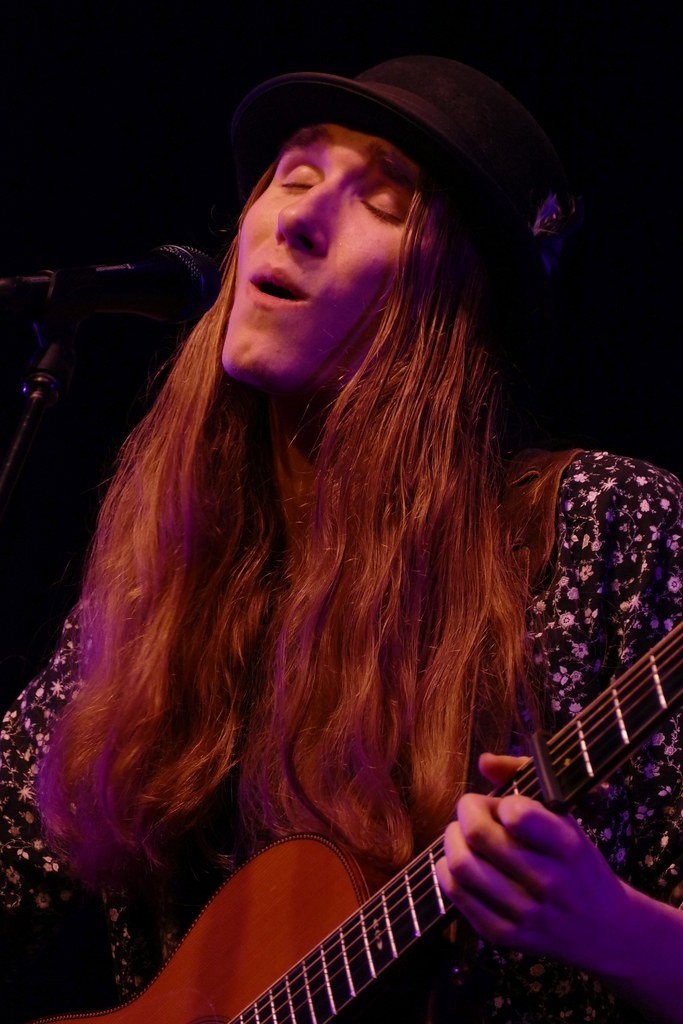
[231,55,568,274]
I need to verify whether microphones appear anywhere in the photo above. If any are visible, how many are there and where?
[0,245,226,324]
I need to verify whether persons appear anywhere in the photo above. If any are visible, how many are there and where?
[0,56,683,1024]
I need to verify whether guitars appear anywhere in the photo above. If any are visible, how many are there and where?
[32,620,683,1023]
